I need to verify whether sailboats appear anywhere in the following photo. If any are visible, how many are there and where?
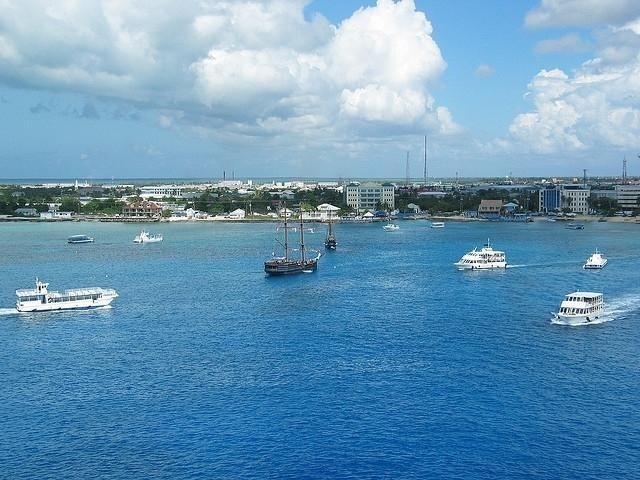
[325,216,337,250]
[264,198,318,276]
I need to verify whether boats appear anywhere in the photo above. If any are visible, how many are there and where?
[453,237,509,271]
[15,277,120,312]
[133,230,164,243]
[430,221,445,228]
[382,222,400,232]
[67,235,95,243]
[555,287,605,324]
[584,247,607,269]
